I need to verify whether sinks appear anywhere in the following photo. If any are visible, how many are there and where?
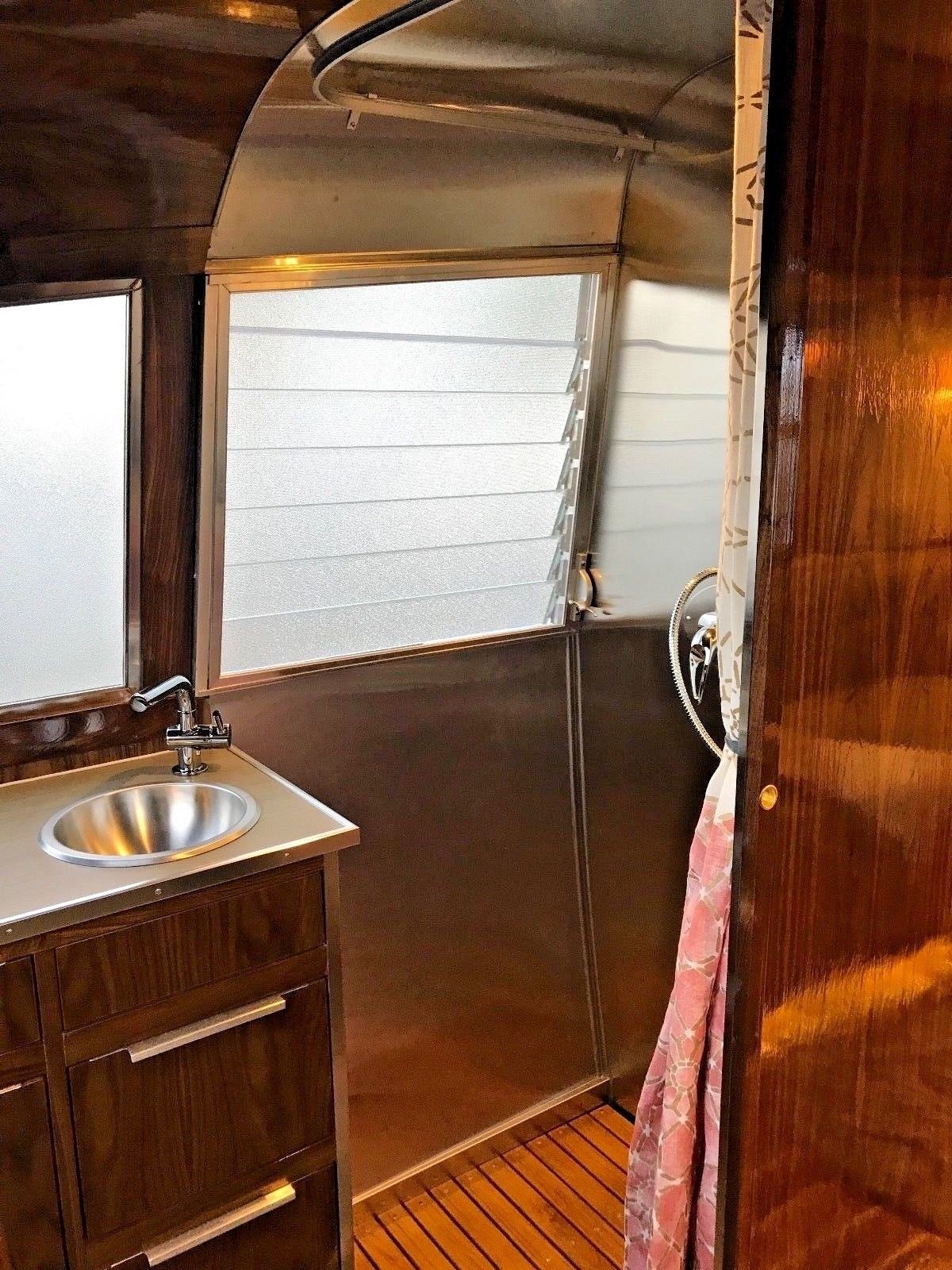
[37,780,262,867]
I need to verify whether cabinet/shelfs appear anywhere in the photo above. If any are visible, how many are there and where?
[0,847,357,1270]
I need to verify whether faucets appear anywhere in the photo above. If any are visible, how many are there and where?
[129,675,232,777]
